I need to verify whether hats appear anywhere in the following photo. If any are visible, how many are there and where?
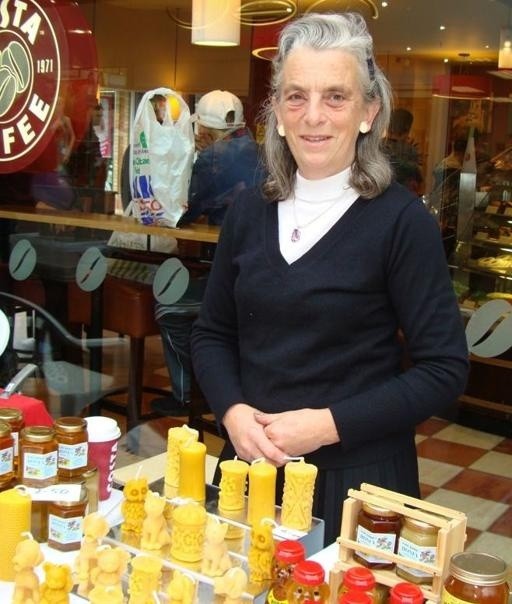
[190,89,247,130]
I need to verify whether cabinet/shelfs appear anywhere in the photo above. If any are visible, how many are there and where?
[404,133,511,441]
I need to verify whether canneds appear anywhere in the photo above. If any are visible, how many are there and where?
[352,500,401,571]
[397,517,444,585]
[0,407,99,553]
[441,555,511,602]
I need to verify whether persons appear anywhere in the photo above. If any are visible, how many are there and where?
[381,107,421,185]
[54,96,79,187]
[71,106,108,213]
[150,89,269,421]
[433,127,470,185]
[120,95,166,211]
[207,12,472,548]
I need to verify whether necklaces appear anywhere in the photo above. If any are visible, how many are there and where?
[286,188,346,243]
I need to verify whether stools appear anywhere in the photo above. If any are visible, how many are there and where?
[66,261,193,454]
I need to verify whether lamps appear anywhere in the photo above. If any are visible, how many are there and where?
[432,52,495,99]
[250,0,301,61]
[190,1,241,46]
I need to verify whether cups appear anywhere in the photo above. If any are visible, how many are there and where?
[83,416,123,500]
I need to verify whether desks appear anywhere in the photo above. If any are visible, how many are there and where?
[31,183,117,215]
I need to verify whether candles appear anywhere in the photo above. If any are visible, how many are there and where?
[498,42,512,68]
